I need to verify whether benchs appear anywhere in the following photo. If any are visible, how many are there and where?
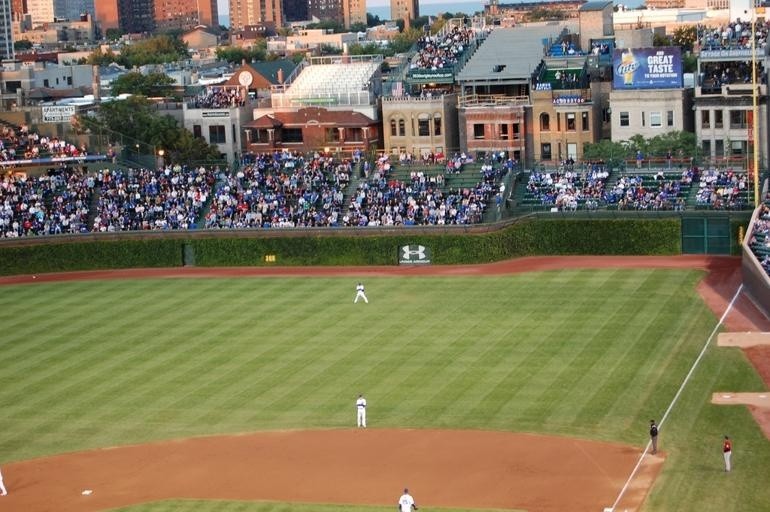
[0,120,67,211]
[751,200,770,272]
[408,44,472,73]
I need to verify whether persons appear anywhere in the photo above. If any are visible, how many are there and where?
[356,395,367,428]
[1,148,515,240]
[555,40,610,89]
[195,87,245,108]
[750,191,770,265]
[416,25,476,72]
[405,88,450,98]
[0,472,7,496]
[526,150,748,211]
[399,488,416,512]
[721,436,731,472]
[353,282,369,304]
[1,123,87,161]
[649,420,657,455]
[673,18,770,89]
